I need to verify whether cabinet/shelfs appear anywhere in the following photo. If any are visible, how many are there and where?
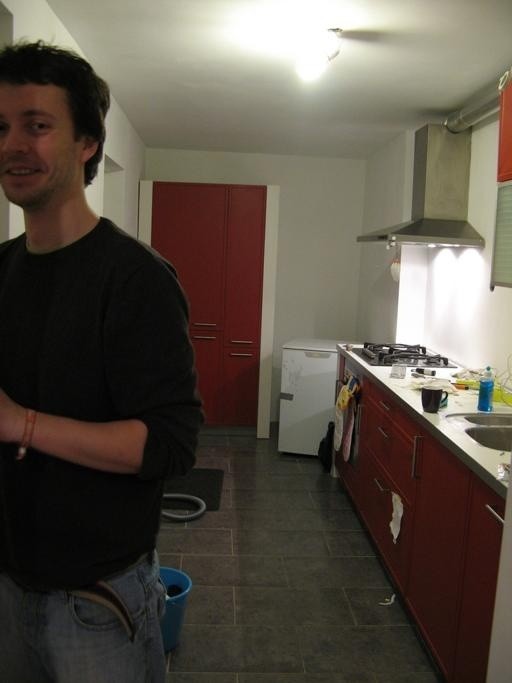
[139,180,282,439]
[360,378,427,596]
[402,436,505,683]
[334,356,375,493]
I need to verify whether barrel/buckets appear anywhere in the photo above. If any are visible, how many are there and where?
[155,565,193,656]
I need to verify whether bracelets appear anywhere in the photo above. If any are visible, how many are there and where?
[16,408,37,460]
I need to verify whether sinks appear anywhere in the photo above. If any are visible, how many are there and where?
[464,429,512,453]
[444,412,512,429]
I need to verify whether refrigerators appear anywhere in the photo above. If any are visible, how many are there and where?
[278,340,345,456]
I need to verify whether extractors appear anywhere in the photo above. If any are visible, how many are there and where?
[357,123,485,250]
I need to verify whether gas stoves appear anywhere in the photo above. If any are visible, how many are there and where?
[352,342,459,368]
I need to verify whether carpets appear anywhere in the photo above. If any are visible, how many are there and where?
[161,468,224,512]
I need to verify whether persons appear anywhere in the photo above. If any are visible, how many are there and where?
[2,38,202,680]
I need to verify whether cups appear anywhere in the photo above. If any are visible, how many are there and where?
[421,384,448,413]
[391,364,407,379]
